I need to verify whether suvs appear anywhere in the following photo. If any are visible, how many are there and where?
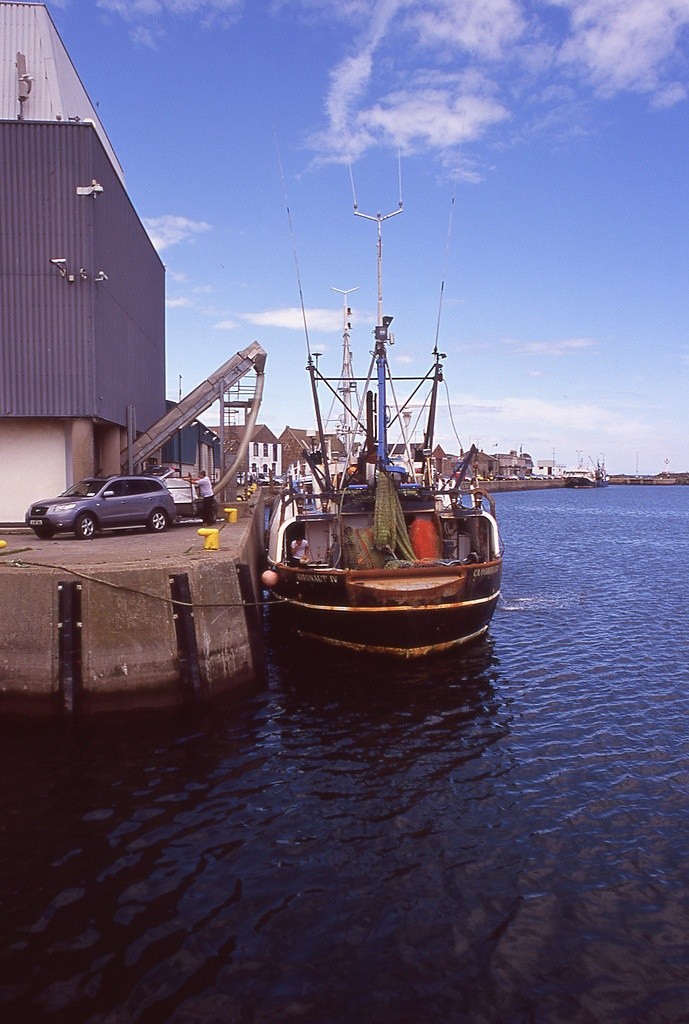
[25,464,177,540]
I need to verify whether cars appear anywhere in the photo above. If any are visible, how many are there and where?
[236,471,282,486]
[455,471,562,480]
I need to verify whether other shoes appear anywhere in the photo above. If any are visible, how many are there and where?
[202,521,213,526]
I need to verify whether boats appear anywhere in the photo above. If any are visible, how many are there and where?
[562,449,611,490]
[262,144,506,669]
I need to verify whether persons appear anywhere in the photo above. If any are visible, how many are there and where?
[290,534,309,569]
[188,470,216,527]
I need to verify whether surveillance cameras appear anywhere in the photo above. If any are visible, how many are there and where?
[50,259,67,264]
[99,271,108,280]
[94,186,104,195]
